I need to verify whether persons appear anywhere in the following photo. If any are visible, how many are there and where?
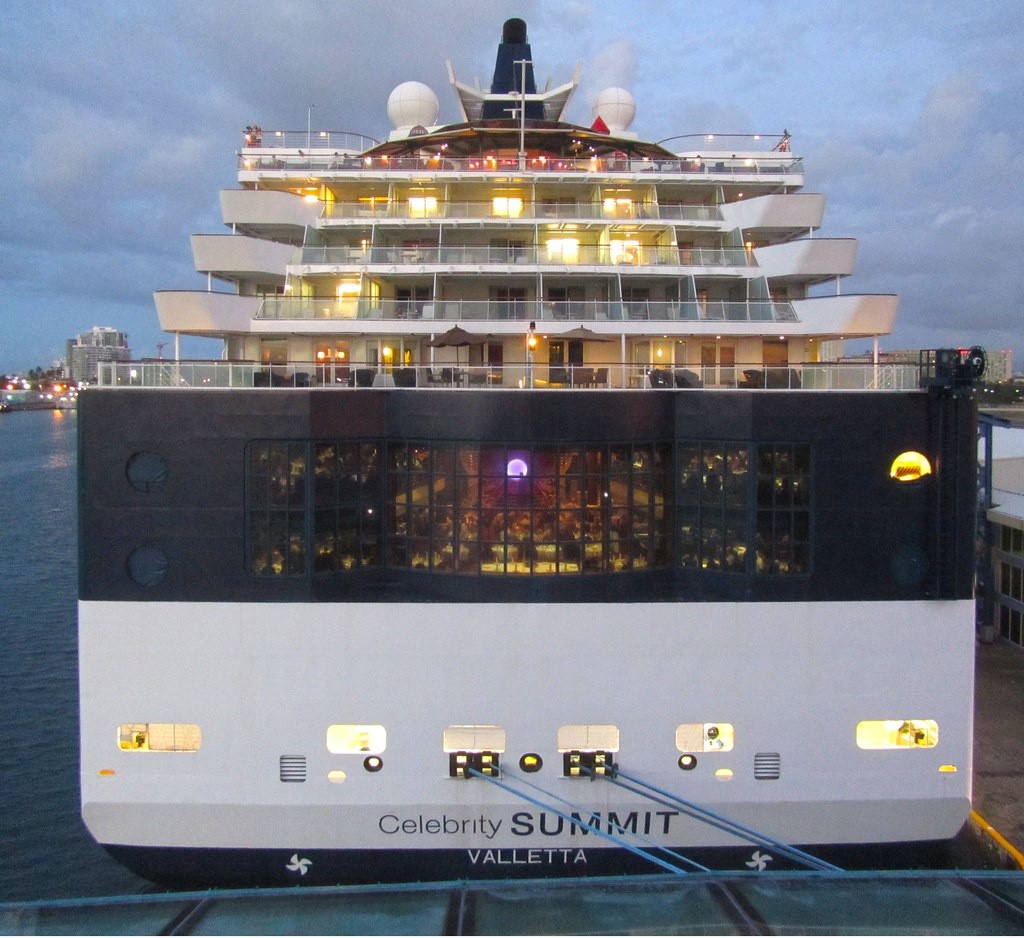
[245,125,262,148]
[269,150,702,172]
[250,448,805,577]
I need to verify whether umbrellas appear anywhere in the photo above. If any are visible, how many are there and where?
[547,325,616,343]
[426,324,486,387]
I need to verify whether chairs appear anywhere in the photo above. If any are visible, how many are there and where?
[253,366,803,390]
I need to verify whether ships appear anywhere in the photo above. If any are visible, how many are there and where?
[74,14,1024,936]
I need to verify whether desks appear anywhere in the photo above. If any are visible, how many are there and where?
[410,543,648,574]
